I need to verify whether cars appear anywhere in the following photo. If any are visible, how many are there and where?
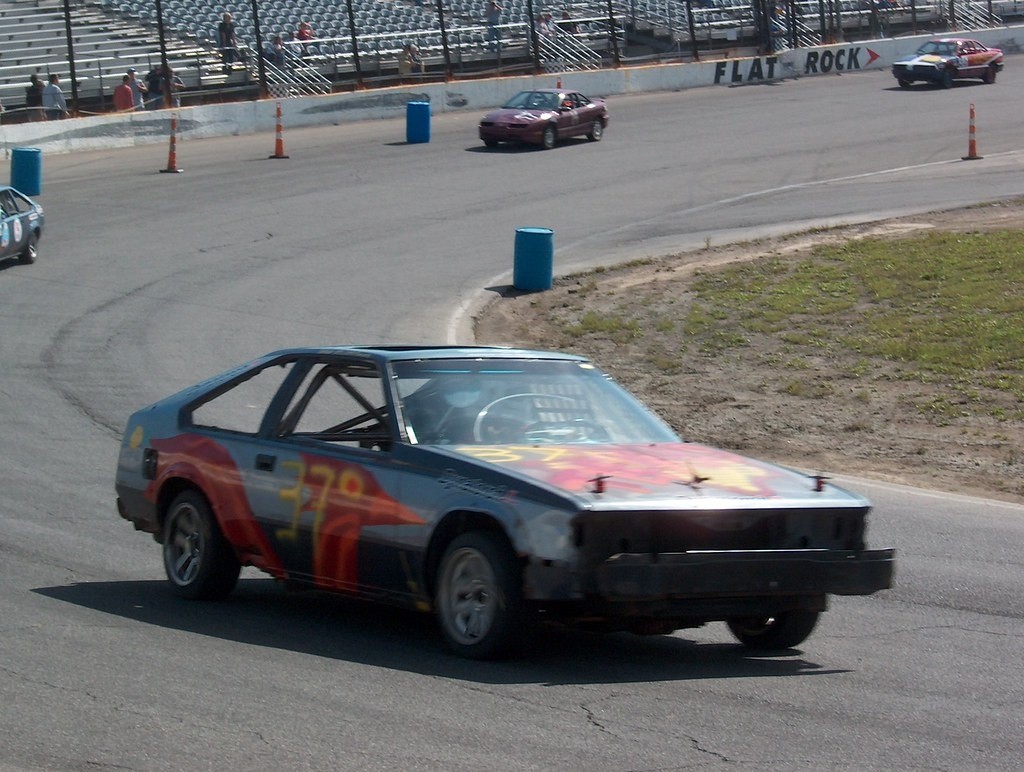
[892,39,1004,89]
[479,90,613,150]
[115,344,899,667]
[0,185,45,265]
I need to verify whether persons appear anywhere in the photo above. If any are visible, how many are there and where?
[26,74,71,122]
[398,43,423,84]
[537,12,581,60]
[219,12,238,75]
[263,21,314,83]
[113,59,185,112]
[484,0,503,53]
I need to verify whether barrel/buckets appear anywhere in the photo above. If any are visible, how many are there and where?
[513,227,556,291]
[9,147,42,196]
[406,101,431,144]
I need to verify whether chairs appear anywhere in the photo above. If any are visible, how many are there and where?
[85,0,899,63]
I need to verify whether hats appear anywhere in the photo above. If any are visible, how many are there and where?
[127,67,136,74]
